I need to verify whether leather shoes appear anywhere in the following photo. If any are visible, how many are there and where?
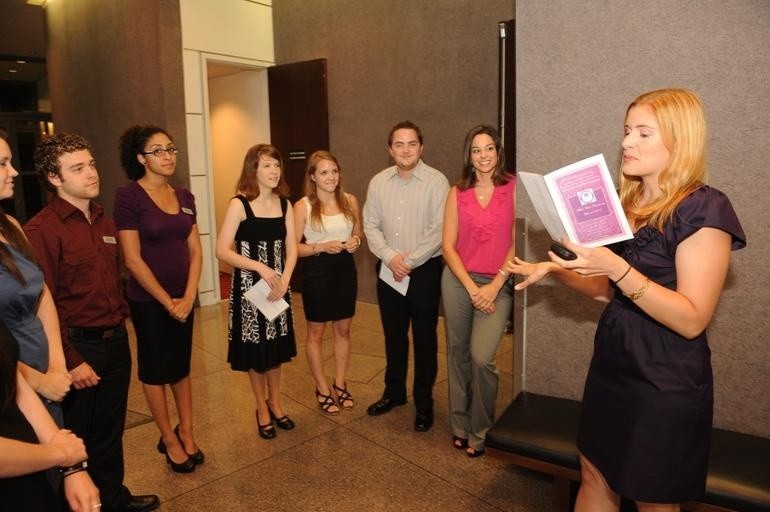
[256,409,275,439]
[127,495,160,512]
[368,395,407,416]
[415,409,433,431]
[266,398,294,430]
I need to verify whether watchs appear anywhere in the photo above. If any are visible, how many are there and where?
[59,460,89,473]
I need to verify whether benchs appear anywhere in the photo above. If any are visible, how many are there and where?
[484,390,770,512]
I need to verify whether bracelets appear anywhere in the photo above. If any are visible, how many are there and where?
[614,264,633,285]
[629,276,654,302]
[312,245,319,258]
[498,267,507,280]
[352,233,362,247]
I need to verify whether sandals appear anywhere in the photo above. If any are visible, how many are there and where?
[333,382,353,410]
[315,386,340,415]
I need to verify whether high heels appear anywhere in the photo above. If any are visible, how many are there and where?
[158,437,195,473]
[175,424,203,464]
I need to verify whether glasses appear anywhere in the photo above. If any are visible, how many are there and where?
[140,147,179,157]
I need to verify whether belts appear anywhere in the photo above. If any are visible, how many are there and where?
[67,320,127,343]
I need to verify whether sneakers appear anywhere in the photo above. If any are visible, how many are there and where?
[453,436,467,448]
[465,445,485,457]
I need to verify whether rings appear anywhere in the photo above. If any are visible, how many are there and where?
[91,503,102,509]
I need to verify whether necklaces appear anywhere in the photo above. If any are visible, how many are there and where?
[474,180,496,204]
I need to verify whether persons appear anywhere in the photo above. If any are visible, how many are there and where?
[504,87,747,512]
[112,123,203,474]
[2,319,103,512]
[1,130,74,487]
[292,148,362,417]
[440,124,518,456]
[214,143,298,439]
[361,120,451,433]
[21,130,160,511]
